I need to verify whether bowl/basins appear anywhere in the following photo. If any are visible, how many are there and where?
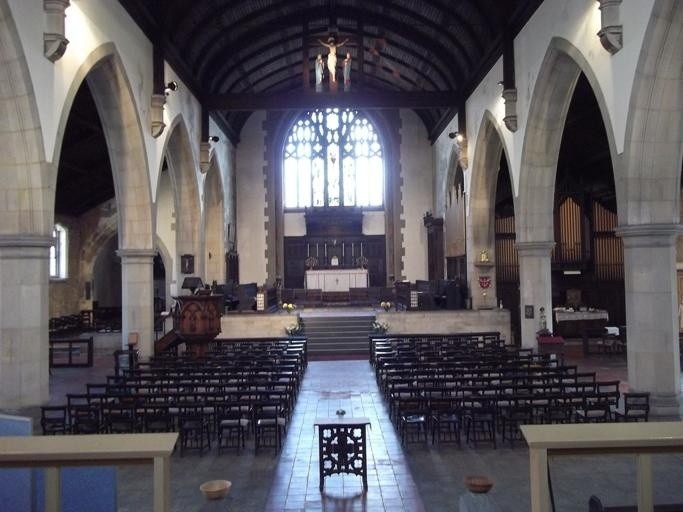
[200,479,232,499]
[465,477,494,493]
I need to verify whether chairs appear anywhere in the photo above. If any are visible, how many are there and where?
[49,306,122,343]
[314,422,371,493]
[624,391,651,421]
[67,336,309,454]
[40,403,67,434]
[366,330,623,448]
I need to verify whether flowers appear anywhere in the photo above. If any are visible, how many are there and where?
[283,303,297,314]
[380,300,393,312]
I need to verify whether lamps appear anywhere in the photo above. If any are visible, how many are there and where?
[209,136,221,145]
[447,131,465,141]
[164,81,180,97]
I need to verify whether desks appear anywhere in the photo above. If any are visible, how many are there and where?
[0,432,182,512]
[520,421,683,512]
[537,334,566,367]
[112,349,139,383]
